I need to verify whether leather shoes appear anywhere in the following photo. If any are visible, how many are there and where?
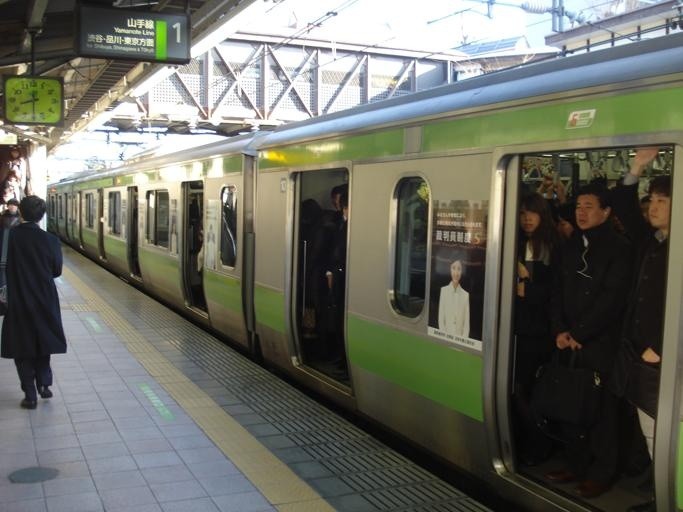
[546,466,578,484]
[37,384,53,397]
[573,478,613,498]
[20,398,38,409]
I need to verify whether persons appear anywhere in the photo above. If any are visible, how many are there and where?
[437,251,470,339]
[0,198,19,225]
[509,147,672,496]
[306,183,348,375]
[0,194,67,407]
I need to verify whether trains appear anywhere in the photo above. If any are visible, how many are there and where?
[45,30,683,511]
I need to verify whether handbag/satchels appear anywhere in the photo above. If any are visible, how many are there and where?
[625,361,659,419]
[0,283,9,317]
